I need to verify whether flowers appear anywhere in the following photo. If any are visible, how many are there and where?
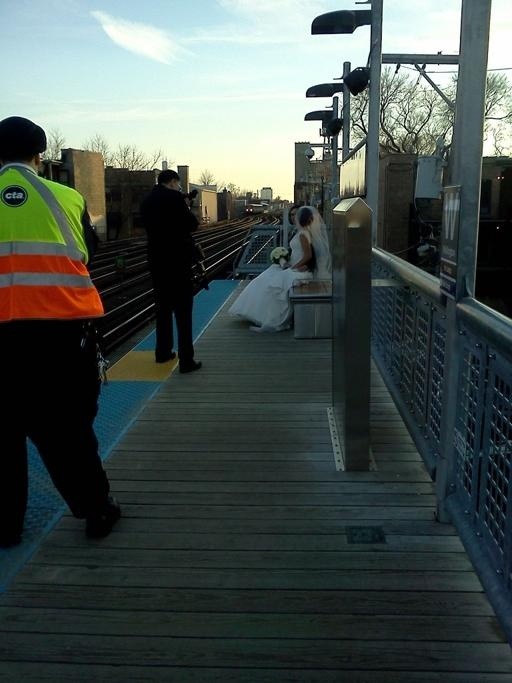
[270,247,290,269]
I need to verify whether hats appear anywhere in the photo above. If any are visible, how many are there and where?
[0,115,47,163]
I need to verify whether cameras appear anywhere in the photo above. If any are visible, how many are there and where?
[180,188,198,203]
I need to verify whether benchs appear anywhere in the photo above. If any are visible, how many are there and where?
[287,278,338,338]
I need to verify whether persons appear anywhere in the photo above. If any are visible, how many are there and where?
[0,114,126,551]
[141,168,203,372]
[228,202,321,333]
[284,205,317,273]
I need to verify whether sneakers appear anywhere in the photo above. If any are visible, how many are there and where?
[84,494,122,540]
[178,361,202,373]
[156,351,176,365]
